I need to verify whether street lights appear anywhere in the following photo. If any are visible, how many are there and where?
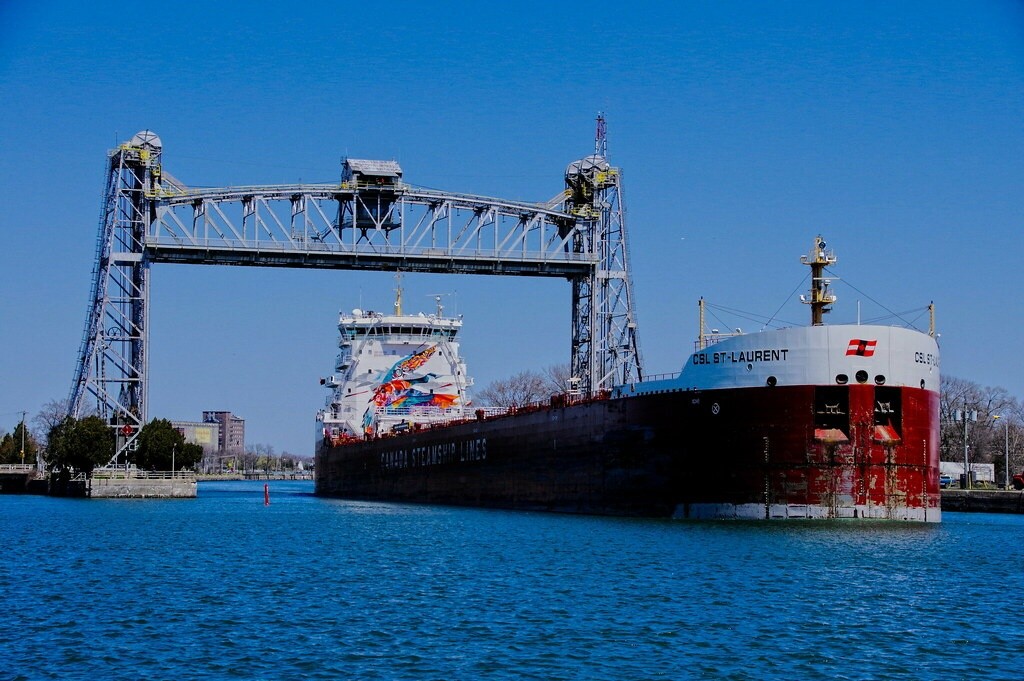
[18,410,29,464]
[993,413,1009,489]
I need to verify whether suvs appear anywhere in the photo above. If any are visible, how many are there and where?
[1013,471,1024,490]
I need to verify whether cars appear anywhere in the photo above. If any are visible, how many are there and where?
[940,471,951,488]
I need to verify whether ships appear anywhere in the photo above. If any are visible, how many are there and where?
[312,267,943,528]
